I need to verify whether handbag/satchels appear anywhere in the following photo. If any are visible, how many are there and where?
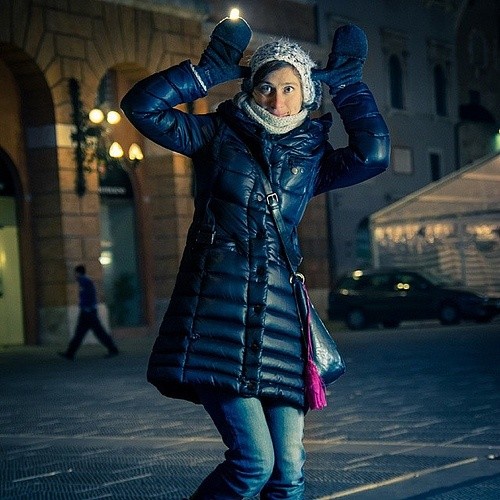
[294,281,345,387]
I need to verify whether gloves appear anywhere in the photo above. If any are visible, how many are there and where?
[310,24,369,96]
[192,17,252,91]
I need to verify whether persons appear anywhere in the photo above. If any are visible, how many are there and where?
[119,16,392,500]
[55,265,121,361]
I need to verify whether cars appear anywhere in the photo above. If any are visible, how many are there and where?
[326,270,490,331]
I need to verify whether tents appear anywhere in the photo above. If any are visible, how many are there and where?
[370,150,500,298]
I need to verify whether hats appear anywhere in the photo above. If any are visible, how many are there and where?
[240,40,323,112]
[74,265,87,276]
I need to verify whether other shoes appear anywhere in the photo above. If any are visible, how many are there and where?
[104,348,119,359]
[56,351,71,360]
